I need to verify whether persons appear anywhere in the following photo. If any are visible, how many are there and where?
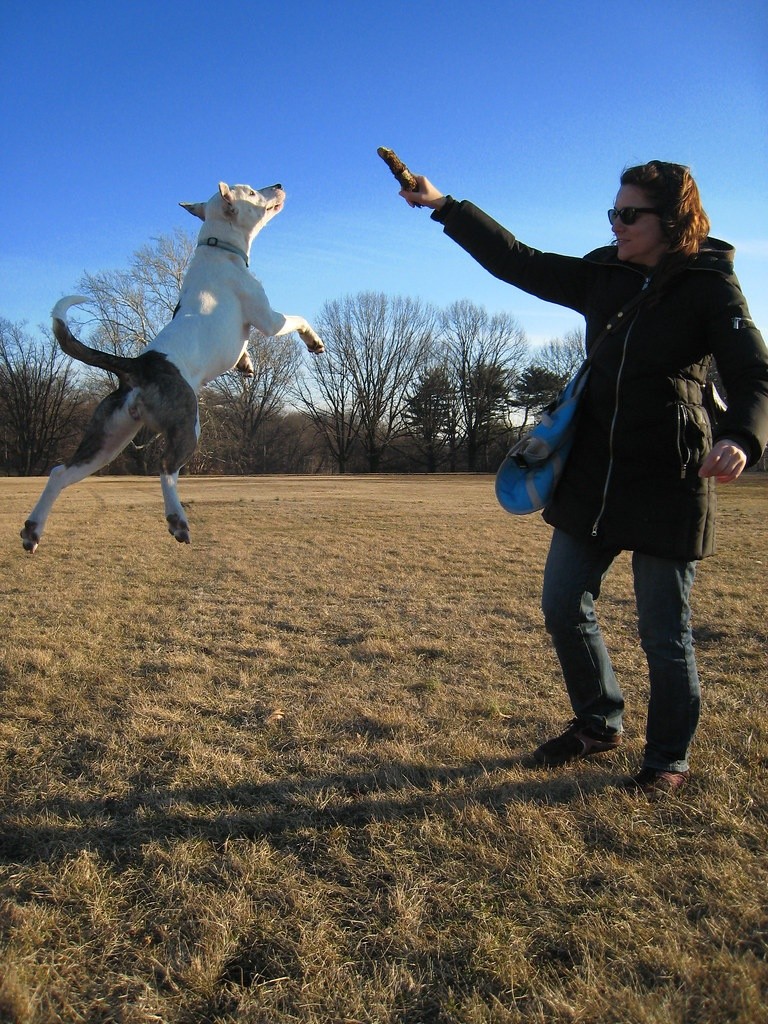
[399,161,768,802]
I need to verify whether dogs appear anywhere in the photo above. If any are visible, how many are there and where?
[20,180,325,553]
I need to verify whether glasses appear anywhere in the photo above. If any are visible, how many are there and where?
[606,205,661,225]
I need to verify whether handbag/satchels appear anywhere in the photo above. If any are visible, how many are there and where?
[496,362,591,515]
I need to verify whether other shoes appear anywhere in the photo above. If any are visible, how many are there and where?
[626,768,694,802]
[534,718,622,766]
